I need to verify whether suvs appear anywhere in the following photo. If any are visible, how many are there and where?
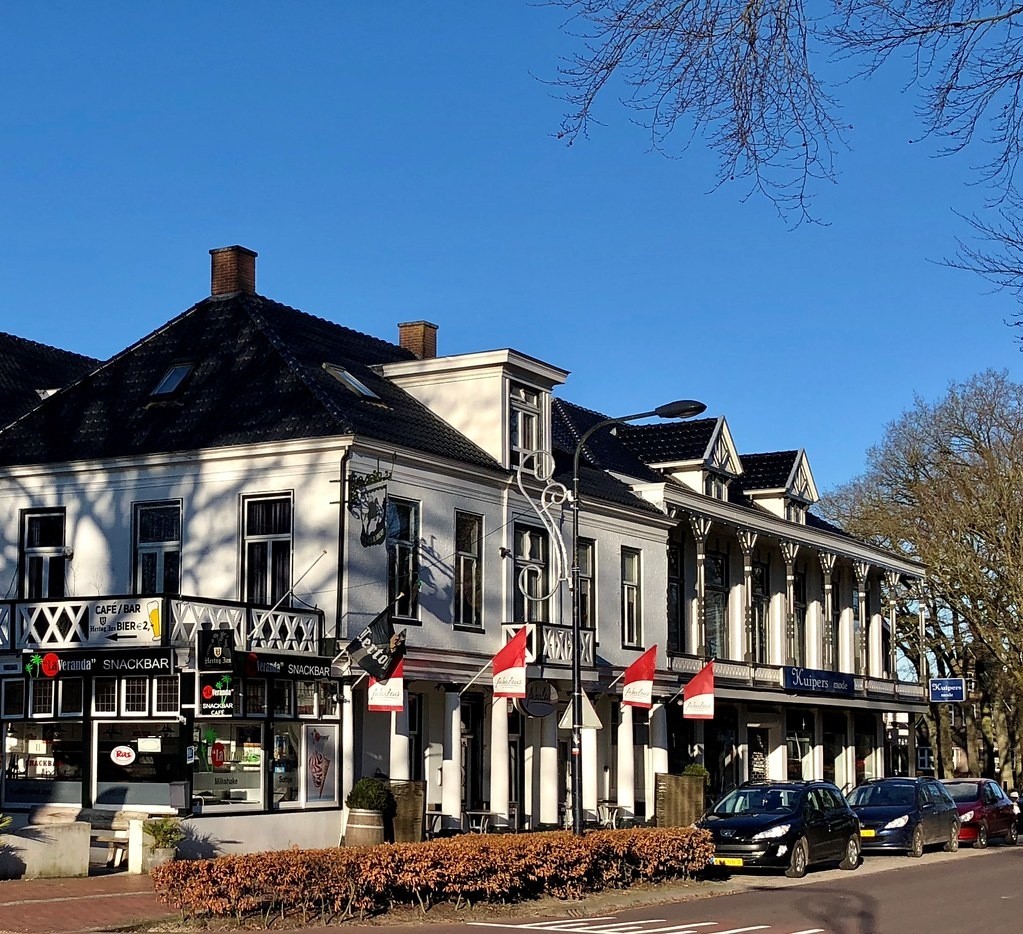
[845,777,961,857]
[692,779,863,878]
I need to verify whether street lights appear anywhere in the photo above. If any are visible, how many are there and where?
[574,401,707,836]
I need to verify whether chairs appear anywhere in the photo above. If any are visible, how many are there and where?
[745,796,766,813]
[4,752,32,779]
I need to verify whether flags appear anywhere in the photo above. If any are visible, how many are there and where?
[368,658,403,711]
[492,626,526,698]
[622,643,657,708]
[683,658,716,719]
[346,597,409,685]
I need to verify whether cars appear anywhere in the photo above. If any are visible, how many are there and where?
[937,778,1020,847]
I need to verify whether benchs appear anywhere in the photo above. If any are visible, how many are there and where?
[582,818,597,829]
[616,815,635,829]
[27,804,150,872]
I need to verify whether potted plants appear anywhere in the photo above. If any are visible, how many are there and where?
[344,777,389,846]
[141,815,184,869]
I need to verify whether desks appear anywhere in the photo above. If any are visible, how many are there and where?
[603,804,632,830]
[425,807,510,843]
